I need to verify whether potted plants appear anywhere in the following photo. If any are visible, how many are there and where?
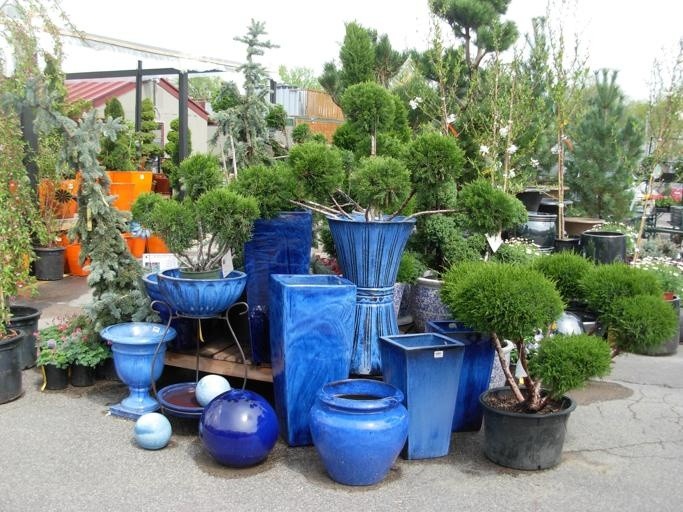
[395,250,416,318]
[0,99,48,404]
[231,80,528,378]
[31,132,66,281]
[441,248,678,470]
[37,335,79,390]
[655,267,679,300]
[412,174,486,333]
[70,332,111,386]
[130,151,279,279]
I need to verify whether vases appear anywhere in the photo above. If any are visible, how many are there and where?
[555,234,580,254]
[564,217,606,236]
[538,198,574,215]
[269,273,356,448]
[517,211,558,247]
[157,382,234,424]
[635,295,681,356]
[425,320,496,434]
[516,186,545,211]
[142,272,209,350]
[308,379,409,486]
[245,207,313,363]
[158,268,247,318]
[540,186,570,199]
[581,231,626,264]
[2,306,42,370]
[531,246,554,255]
[378,333,465,461]
[100,321,176,413]
